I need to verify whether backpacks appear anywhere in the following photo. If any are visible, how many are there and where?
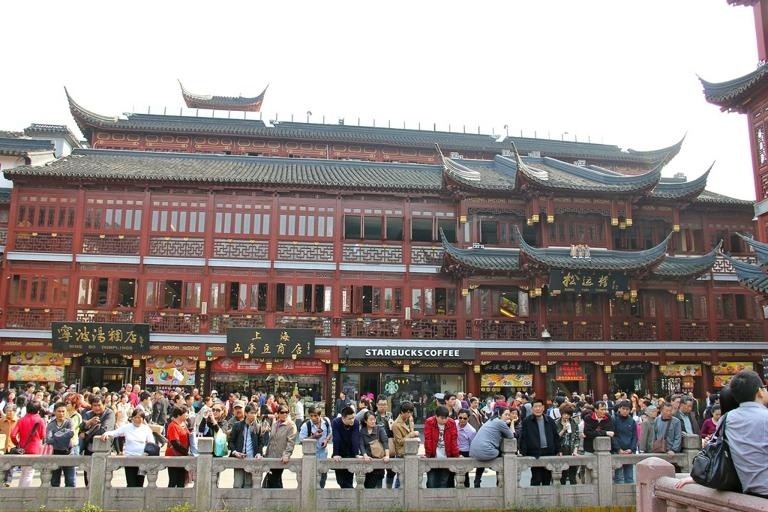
[299,418,329,439]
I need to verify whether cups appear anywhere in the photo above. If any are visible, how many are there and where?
[565,423,571,434]
[187,379,192,383]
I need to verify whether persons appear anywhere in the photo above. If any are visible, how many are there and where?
[0,382,737,489]
[674,371,768,498]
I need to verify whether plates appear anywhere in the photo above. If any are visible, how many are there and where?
[10,365,49,381]
[726,366,733,373]
[679,368,686,377]
[11,351,58,365]
[714,367,722,373]
[720,382,730,387]
[735,365,743,371]
[689,368,696,376]
[146,356,184,384]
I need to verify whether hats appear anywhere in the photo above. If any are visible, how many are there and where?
[232,400,244,408]
[58,383,68,388]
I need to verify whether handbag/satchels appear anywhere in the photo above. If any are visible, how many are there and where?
[691,411,738,490]
[189,432,203,457]
[368,425,396,457]
[653,418,671,453]
[213,423,228,456]
[10,447,25,454]
[262,472,272,488]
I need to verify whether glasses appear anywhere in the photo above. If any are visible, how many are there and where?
[459,417,467,420]
[760,385,768,390]
[212,408,220,413]
[280,411,289,414]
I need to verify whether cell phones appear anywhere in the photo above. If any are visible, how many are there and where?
[208,409,212,415]
[318,429,324,434]
